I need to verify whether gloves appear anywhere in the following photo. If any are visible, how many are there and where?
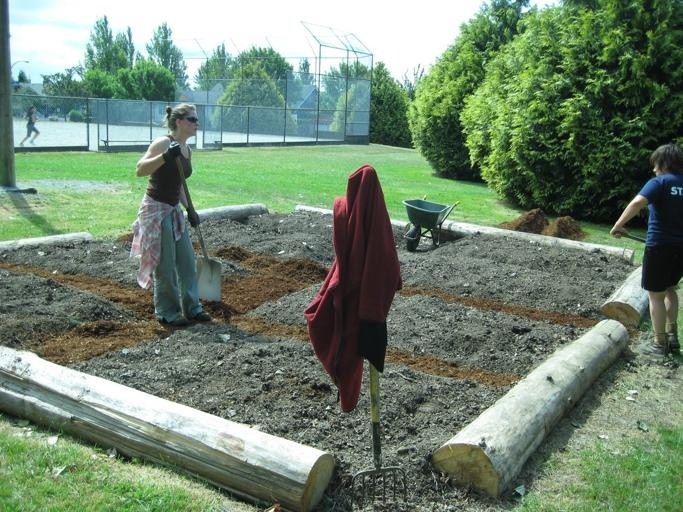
[162,141,182,163]
[187,206,200,228]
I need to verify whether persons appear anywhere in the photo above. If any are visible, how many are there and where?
[610,141,683,356]
[133,102,210,327]
[19,106,40,147]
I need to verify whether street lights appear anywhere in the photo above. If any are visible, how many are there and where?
[11,60,29,70]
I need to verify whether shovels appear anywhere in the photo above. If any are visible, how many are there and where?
[170,137,222,302]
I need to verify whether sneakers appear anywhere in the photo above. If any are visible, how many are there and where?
[639,341,681,355]
[185,310,211,321]
[159,315,192,326]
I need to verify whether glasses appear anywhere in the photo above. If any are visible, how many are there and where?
[181,117,199,123]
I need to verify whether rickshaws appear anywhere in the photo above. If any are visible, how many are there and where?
[401,194,460,252]
